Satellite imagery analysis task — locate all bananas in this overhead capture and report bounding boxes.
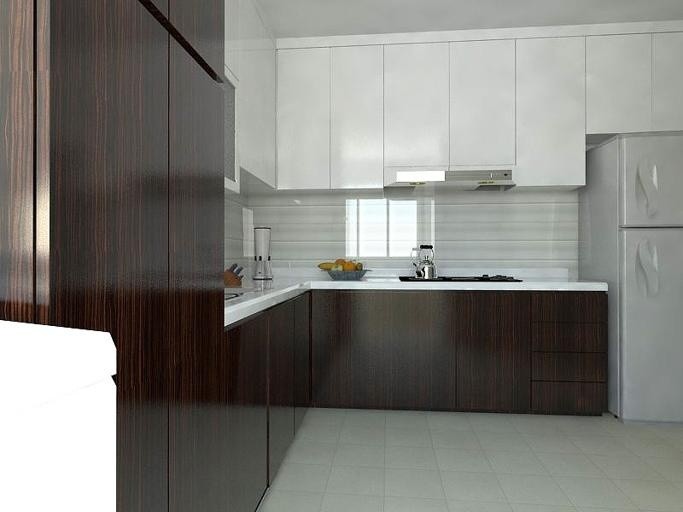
[318,263,335,270]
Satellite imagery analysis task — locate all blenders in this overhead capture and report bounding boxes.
[252,226,274,280]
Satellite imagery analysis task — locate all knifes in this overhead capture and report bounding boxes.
[228,263,244,280]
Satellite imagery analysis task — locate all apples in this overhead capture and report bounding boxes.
[344,262,353,271]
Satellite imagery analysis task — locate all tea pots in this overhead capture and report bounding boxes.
[413,244,437,280]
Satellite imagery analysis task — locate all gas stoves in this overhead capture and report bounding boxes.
[398,275,524,282]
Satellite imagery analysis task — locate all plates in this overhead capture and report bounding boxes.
[328,270,370,280]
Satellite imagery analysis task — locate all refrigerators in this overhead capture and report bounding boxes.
[576,133,683,425]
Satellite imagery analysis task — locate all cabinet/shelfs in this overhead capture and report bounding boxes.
[275,29,383,195]
[311,289,609,416]
[224,292,310,511]
[224,0,275,196]
[506,25,586,193]
[585,19,683,140]
[383,27,516,168]
[0,0,224,511]
